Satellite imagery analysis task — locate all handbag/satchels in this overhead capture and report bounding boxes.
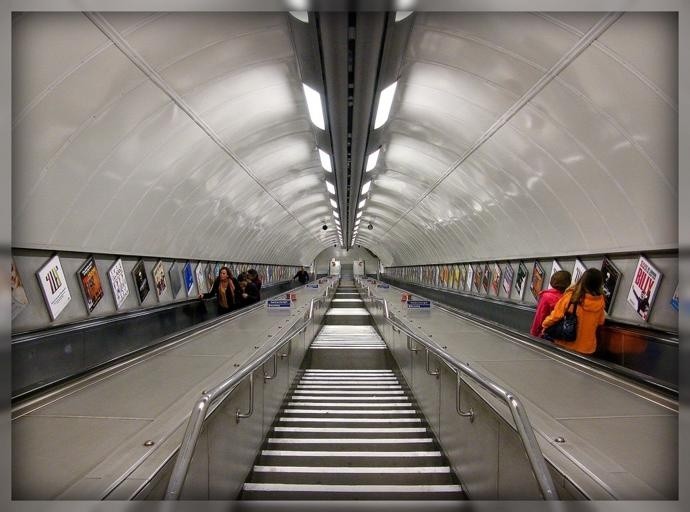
[544,312,577,341]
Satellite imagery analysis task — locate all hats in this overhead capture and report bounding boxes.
[550,271,571,289]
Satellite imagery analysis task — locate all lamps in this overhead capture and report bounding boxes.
[287,10,416,250]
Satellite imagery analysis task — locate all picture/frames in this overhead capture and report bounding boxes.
[383,253,678,327]
[12,248,308,327]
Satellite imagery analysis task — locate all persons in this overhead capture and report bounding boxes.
[529,270,572,340]
[197,267,244,316]
[631,283,651,317]
[11,258,30,305]
[539,267,609,357]
[246,269,262,302]
[235,272,260,307]
[292,266,310,285]
